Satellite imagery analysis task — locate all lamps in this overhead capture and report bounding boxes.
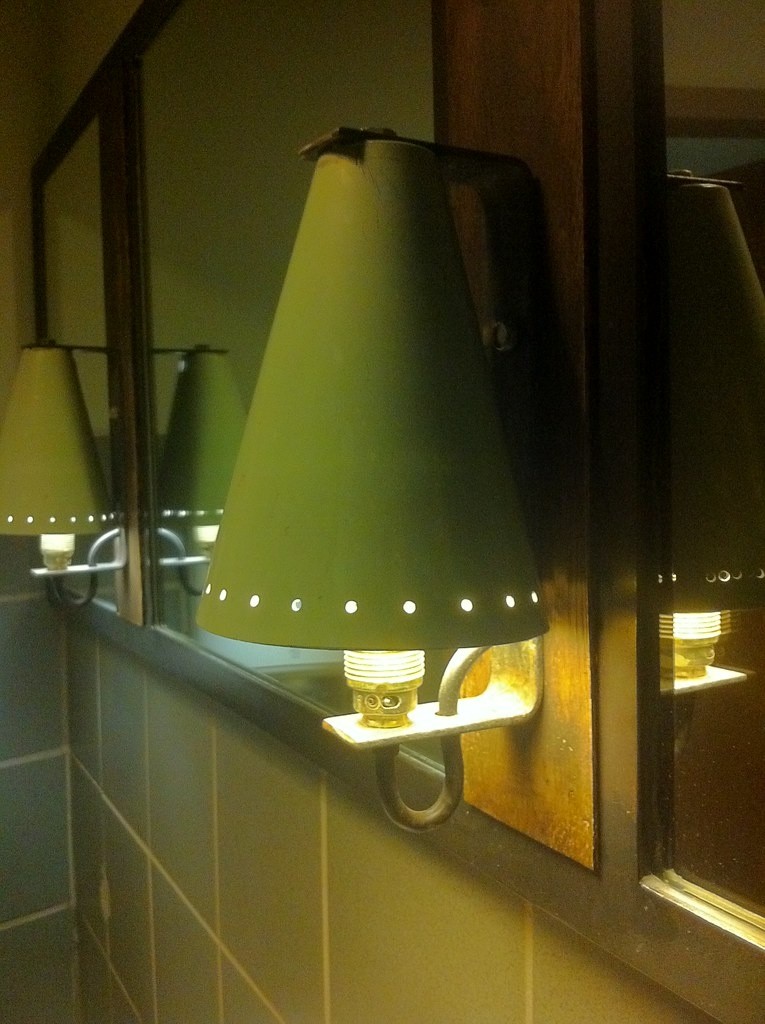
[0,338,127,609]
[156,344,248,597]
[196,124,551,835]
[658,168,765,765]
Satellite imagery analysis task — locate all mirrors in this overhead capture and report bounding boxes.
[31,0,765,1024]
